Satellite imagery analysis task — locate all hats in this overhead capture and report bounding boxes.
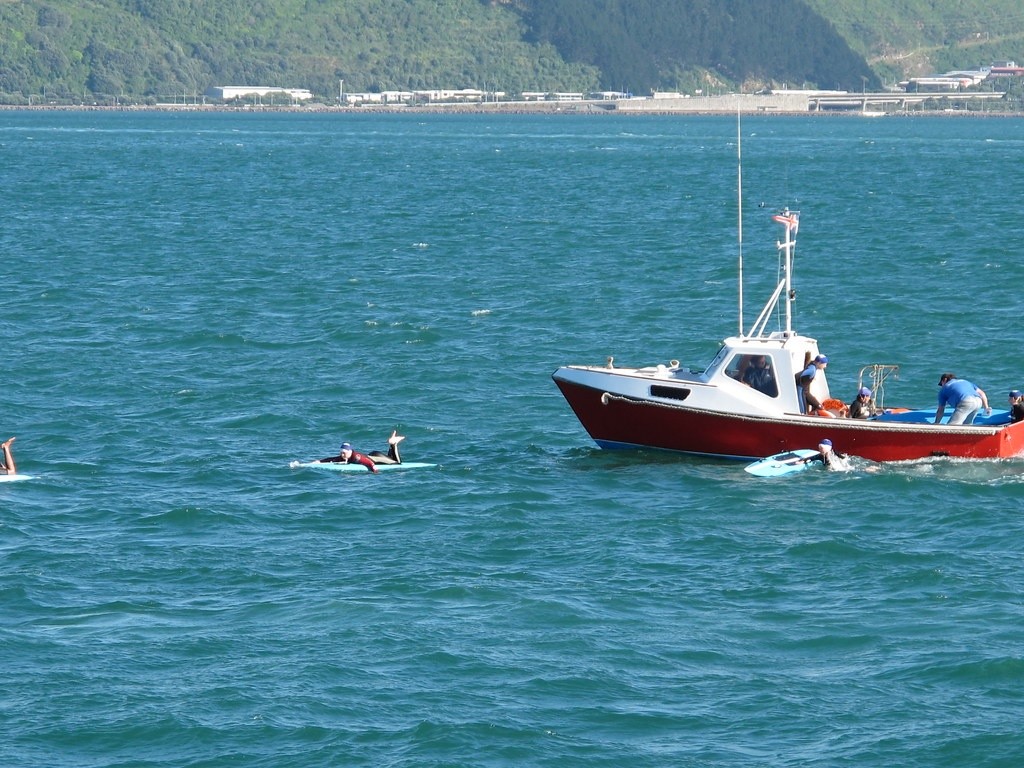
[858,386,871,396]
[1009,390,1022,397]
[815,354,828,363]
[340,443,352,452]
[938,373,950,386]
[819,439,832,450]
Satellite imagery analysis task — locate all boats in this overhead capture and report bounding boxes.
[742,449,850,477]
[549,99,1024,465]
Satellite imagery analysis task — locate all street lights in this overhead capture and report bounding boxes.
[339,79,344,104]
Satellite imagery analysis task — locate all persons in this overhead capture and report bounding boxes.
[795,438,840,468]
[0,436,18,476]
[1008,389,1024,425]
[794,353,836,417]
[848,386,885,420]
[934,373,989,426]
[310,430,405,474]
[742,354,778,398]
